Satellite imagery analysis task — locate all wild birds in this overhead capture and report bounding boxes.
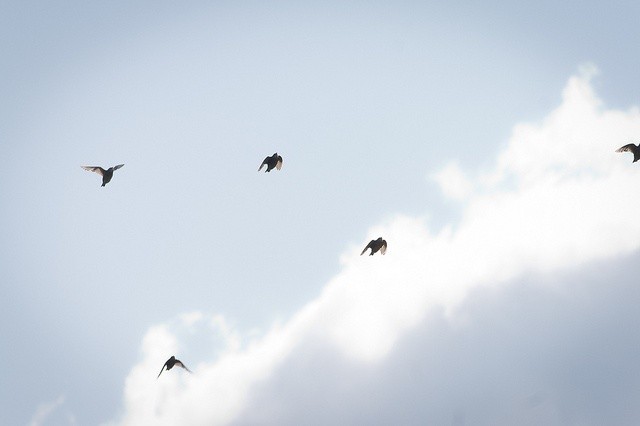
[80,164,127,187]
[615,141,640,165]
[360,236,388,257]
[157,356,192,379]
[256,151,284,174]
